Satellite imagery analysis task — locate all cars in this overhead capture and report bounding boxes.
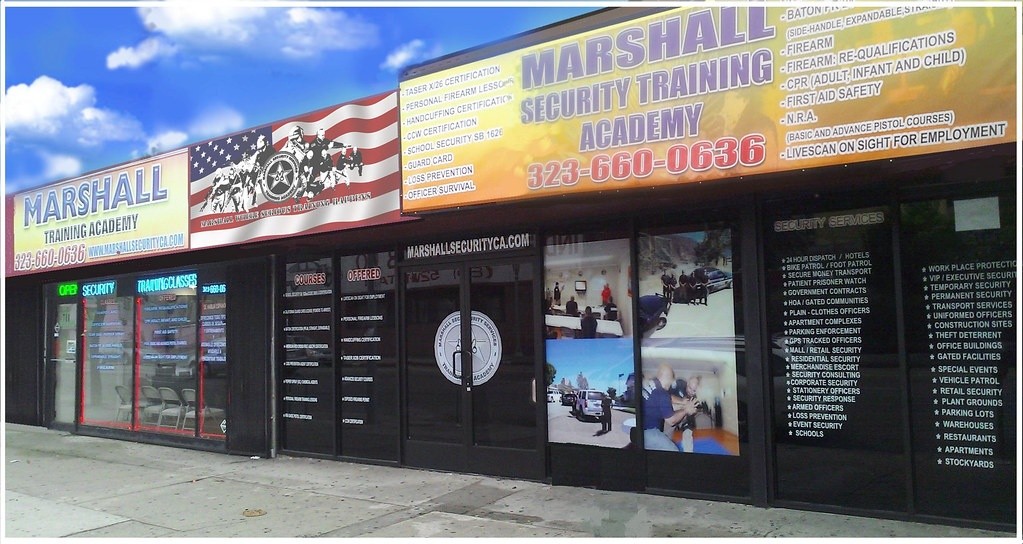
[638,293,672,332]
[189,339,227,377]
[691,267,733,293]
[287,336,317,376]
[547,387,576,405]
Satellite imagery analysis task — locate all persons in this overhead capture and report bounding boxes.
[544,279,619,339]
[600,393,612,430]
[642,363,701,452]
[660,267,711,307]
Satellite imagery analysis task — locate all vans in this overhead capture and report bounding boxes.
[574,390,605,420]
